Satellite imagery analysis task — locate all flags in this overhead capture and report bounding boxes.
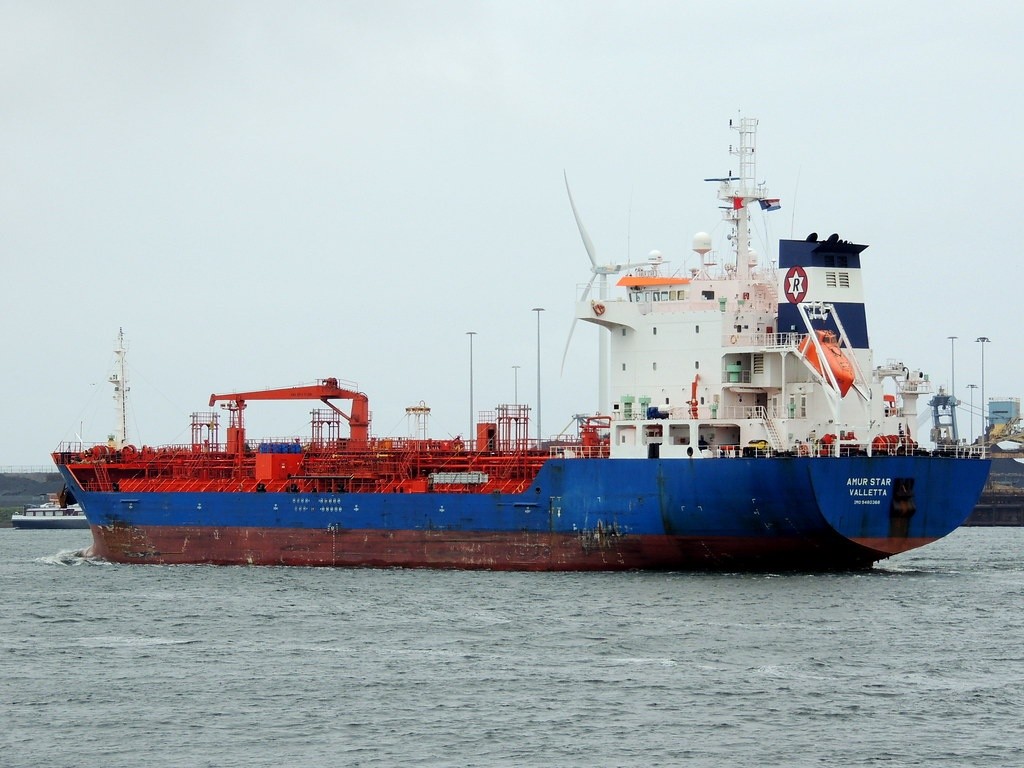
[766,199,781,211]
[734,197,744,210]
[758,200,771,210]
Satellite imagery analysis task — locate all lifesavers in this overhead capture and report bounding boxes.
[595,302,605,315]
[729,333,738,346]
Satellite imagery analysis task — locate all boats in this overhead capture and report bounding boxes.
[923,335,1024,527]
[12,502,90,530]
[49,98,993,578]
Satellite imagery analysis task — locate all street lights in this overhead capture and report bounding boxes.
[966,384,978,442]
[974,336,991,451]
[947,334,957,398]
